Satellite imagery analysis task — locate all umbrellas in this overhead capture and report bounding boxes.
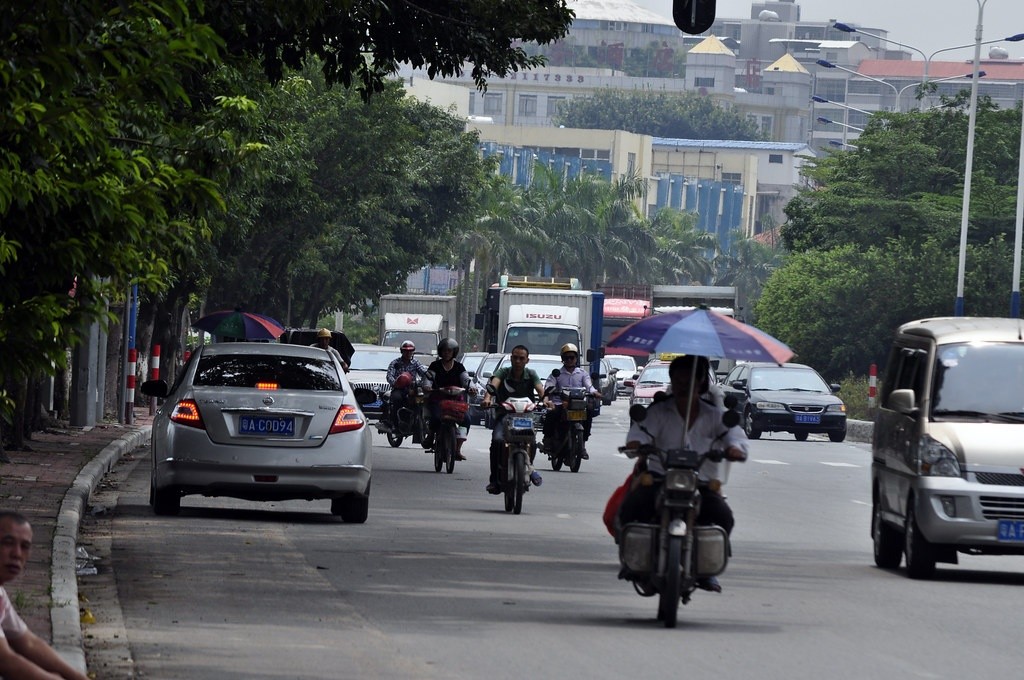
[605,302,799,450]
[189,310,286,341]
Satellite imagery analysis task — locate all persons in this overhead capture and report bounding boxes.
[0,510,92,680]
[309,328,350,372]
[386,339,427,427]
[421,337,479,460]
[603,357,725,547]
[479,345,555,494]
[542,343,602,459]
[618,356,746,593]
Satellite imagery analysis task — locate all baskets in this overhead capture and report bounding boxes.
[437,400,470,426]
[501,412,541,443]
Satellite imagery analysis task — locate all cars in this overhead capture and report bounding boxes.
[623,352,718,409]
[718,362,848,443]
[140,342,372,523]
[346,344,400,418]
[462,352,637,429]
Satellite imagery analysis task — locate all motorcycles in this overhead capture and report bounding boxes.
[617,394,747,628]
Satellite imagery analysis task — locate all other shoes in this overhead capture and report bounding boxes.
[618,567,632,578]
[486,482,499,492]
[531,472,542,486]
[582,449,589,460]
[457,450,466,460]
[700,576,721,593]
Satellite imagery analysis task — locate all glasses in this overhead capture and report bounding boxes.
[564,355,576,360]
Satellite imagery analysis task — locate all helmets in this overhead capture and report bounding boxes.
[317,328,331,337]
[400,341,416,351]
[561,343,578,358]
[438,338,459,358]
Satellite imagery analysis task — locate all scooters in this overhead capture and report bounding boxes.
[376,366,477,474]
[478,386,549,515]
[537,369,605,473]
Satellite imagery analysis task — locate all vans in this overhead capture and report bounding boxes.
[870,316,1024,579]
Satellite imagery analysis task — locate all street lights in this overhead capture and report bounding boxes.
[833,22,1024,114]
[816,60,986,113]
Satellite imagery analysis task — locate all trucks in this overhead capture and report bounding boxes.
[642,285,746,382]
[474,275,651,416]
[376,294,458,356]
